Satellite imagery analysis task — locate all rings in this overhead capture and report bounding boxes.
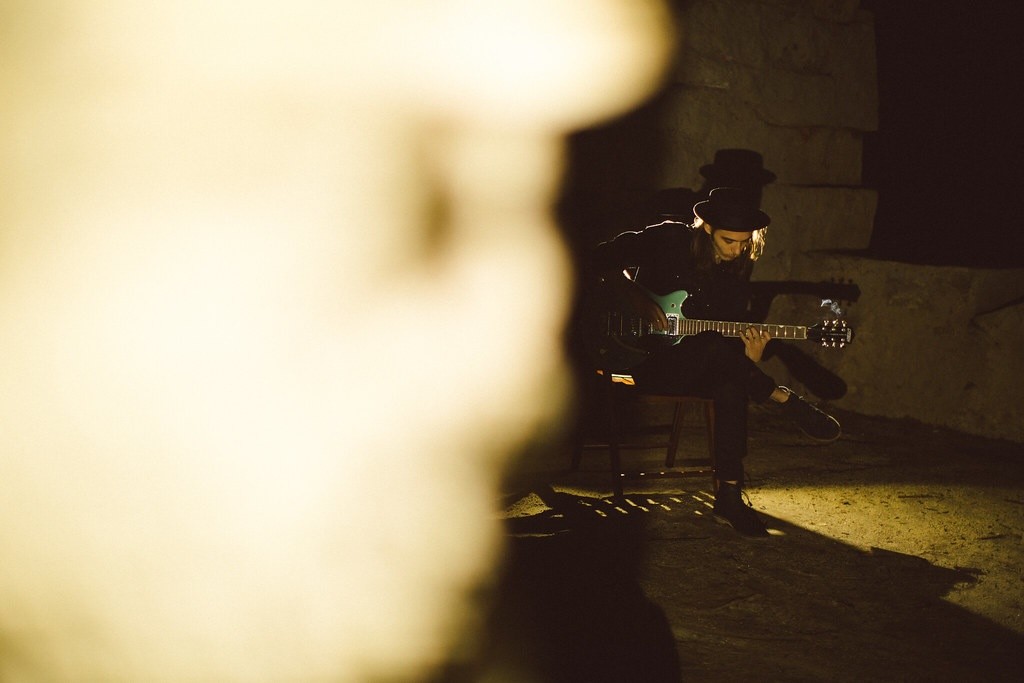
[656,317,660,322]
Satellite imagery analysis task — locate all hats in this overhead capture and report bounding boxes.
[693,187,771,232]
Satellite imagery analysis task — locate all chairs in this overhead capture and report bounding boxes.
[564,370,720,507]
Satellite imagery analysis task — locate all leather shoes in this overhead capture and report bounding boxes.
[714,482,765,531]
[777,386,842,442]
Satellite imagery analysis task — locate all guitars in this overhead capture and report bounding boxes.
[580,276,854,371]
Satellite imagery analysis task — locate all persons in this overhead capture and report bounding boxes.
[594,187,841,534]
[0,0,676,683]
